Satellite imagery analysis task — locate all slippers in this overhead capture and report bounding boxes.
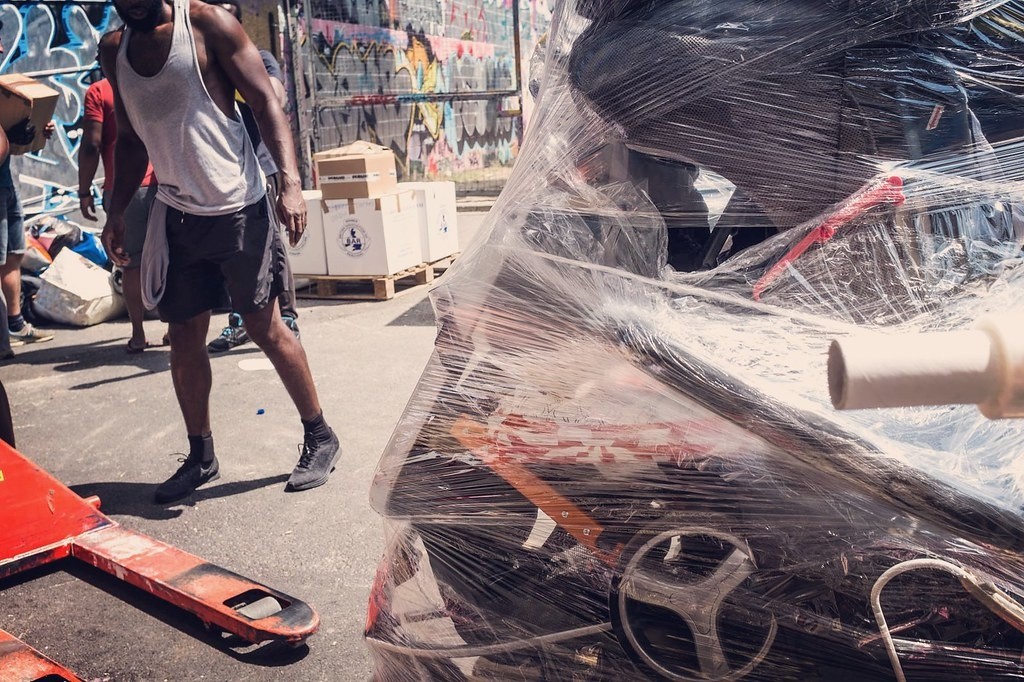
[127,337,149,353]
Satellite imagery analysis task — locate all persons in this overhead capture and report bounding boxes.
[0,18,60,368]
[100,0,341,505]
[79,0,287,349]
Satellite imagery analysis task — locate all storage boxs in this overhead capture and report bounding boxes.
[314,139,392,191]
[276,190,326,274]
[323,189,422,275]
[318,152,398,200]
[0,74,60,155]
[396,180,459,264]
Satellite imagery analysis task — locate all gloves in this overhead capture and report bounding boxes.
[5,115,37,146]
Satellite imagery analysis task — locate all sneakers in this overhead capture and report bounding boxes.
[155,454,218,504]
[8,320,53,347]
[207,313,253,354]
[284,427,342,492]
[281,316,299,333]
